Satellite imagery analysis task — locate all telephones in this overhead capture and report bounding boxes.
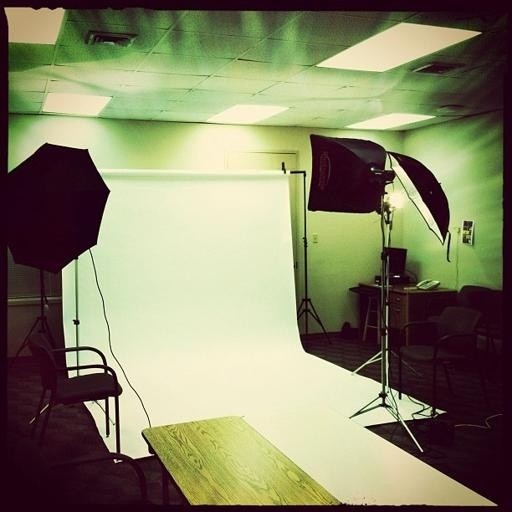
[416,279,441,291]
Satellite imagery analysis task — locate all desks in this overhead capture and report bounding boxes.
[141,417,344,507]
[359,281,458,346]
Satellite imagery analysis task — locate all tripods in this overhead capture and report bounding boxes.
[14,272,58,363]
[297,194,335,354]
[345,225,426,456]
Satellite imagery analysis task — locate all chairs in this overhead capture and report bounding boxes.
[399,285,502,385]
[28,335,122,453]
